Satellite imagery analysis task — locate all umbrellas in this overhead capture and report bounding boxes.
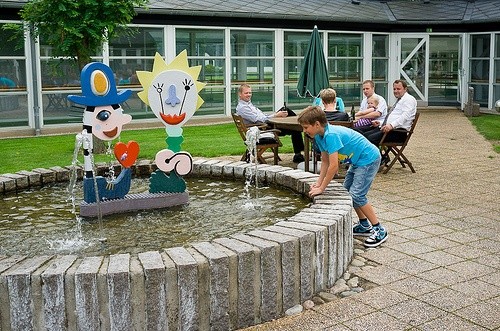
[296,25,330,99]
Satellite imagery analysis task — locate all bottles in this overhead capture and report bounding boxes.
[282,102,287,111]
[336,102,340,110]
[351,103,355,120]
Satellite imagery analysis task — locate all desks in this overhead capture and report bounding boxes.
[267,116,354,173]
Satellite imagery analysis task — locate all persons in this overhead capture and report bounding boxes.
[315,79,416,173]
[0,77,17,89]
[236,83,312,163]
[298,106,388,248]
[113,69,139,85]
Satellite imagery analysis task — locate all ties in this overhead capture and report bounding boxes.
[383,99,399,125]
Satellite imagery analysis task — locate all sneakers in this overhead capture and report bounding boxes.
[353,221,375,236]
[364,226,388,247]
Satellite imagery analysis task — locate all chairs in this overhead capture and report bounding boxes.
[231,112,283,163]
[377,112,421,175]
[302,119,353,180]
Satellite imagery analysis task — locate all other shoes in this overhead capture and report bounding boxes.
[378,156,387,171]
[293,154,304,163]
